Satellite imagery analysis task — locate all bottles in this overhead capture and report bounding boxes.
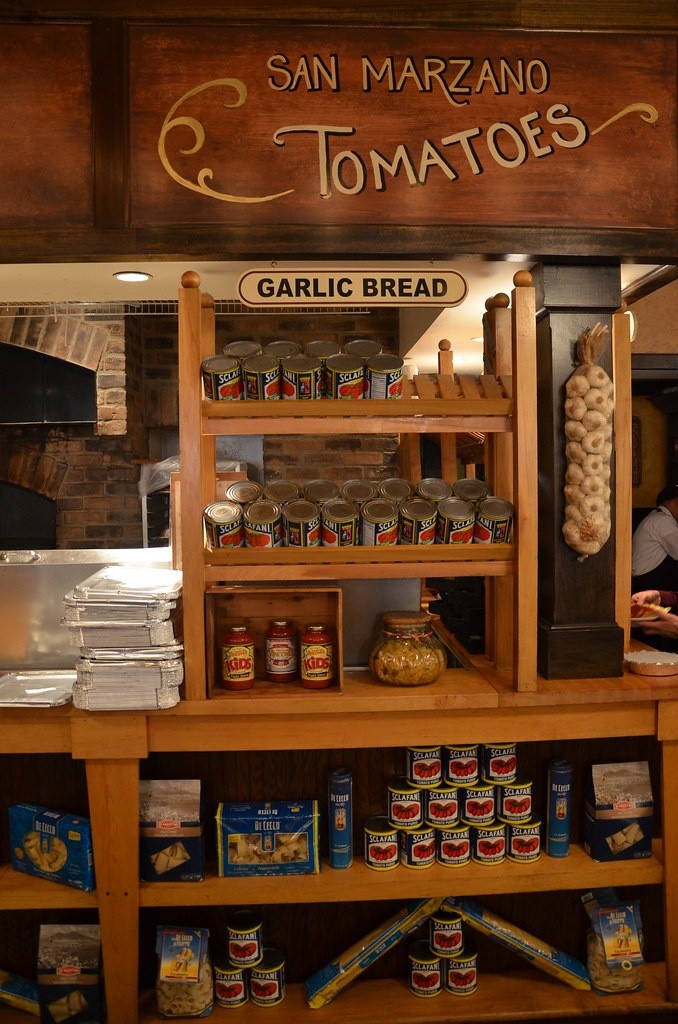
[369,611,447,686]
[543,759,573,858]
[327,766,352,868]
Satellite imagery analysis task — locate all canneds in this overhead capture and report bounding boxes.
[212,912,285,1007]
[264,620,336,688]
[201,339,404,401]
[202,477,515,548]
[220,625,254,689]
[363,741,542,871]
[407,912,477,998]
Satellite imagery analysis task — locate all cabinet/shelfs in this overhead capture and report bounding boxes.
[0,264,676,1024]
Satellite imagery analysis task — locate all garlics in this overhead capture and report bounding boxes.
[561,365,615,555]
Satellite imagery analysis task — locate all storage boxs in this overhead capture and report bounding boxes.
[9,803,95,892]
[216,801,321,877]
[582,761,653,862]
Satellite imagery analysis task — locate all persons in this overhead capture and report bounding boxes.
[631,590,678,640]
[632,486,678,594]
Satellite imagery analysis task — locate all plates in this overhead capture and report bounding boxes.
[631,606,671,627]
